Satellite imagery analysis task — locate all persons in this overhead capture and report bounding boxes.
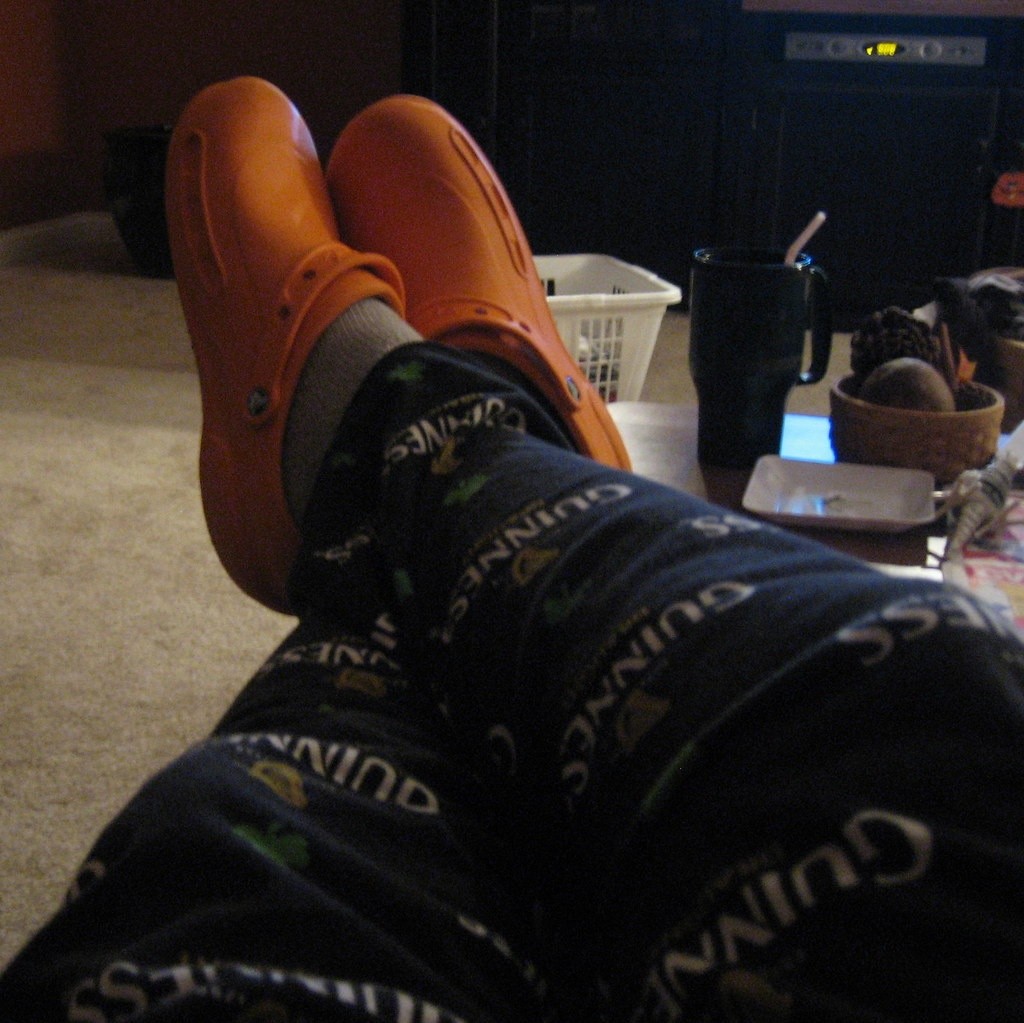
[0,77,1024,1022]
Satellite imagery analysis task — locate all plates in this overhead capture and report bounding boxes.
[741,454,935,534]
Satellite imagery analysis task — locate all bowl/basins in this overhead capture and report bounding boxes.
[827,371,1007,489]
[932,310,1024,436]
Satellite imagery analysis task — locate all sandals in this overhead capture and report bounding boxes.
[324,93,631,474]
[163,74,407,616]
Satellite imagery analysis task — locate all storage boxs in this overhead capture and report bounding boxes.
[533,254,682,408]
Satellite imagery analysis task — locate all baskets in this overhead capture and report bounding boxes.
[530,253,682,402]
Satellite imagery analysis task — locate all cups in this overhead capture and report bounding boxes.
[687,245,833,469]
